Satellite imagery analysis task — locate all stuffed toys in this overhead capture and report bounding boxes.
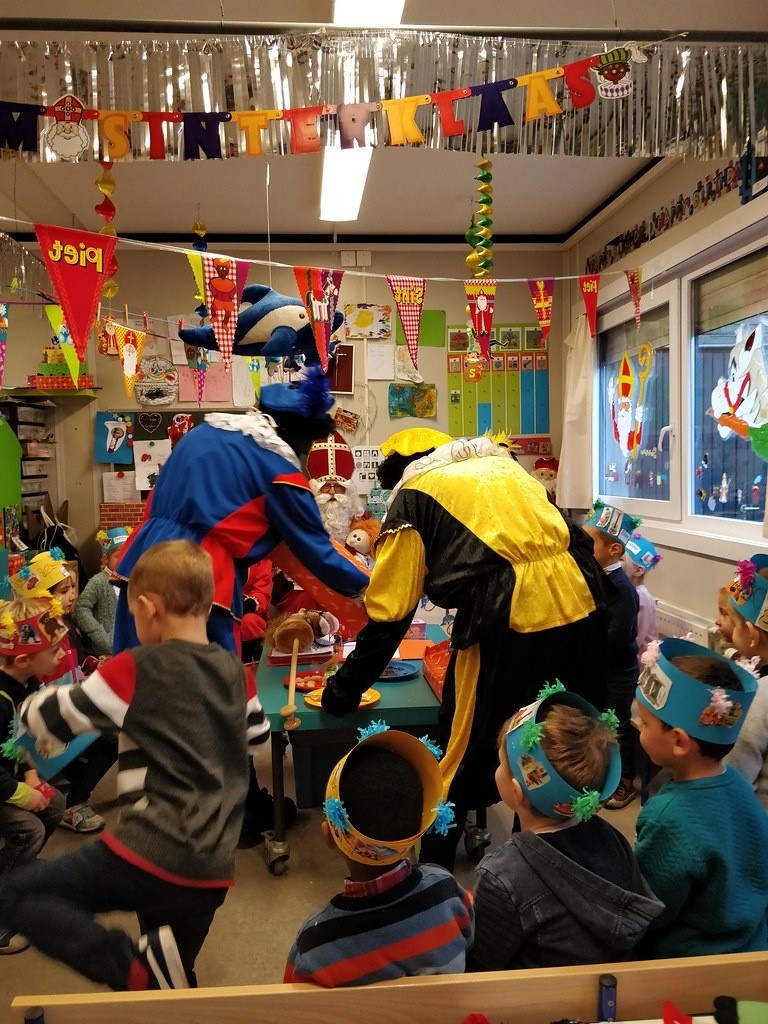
[342,515,384,568]
[178,282,345,357]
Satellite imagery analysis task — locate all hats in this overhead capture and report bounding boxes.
[255,362,336,417]
[379,428,456,456]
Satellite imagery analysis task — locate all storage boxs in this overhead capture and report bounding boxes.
[16,406,54,531]
[27,345,94,390]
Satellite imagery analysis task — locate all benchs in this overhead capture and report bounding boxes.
[9,952,768,1024]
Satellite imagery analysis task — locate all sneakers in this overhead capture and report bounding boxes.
[60,800,106,832]
[605,781,638,808]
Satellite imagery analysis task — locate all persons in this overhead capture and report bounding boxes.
[712,552,768,812]
[627,637,768,962]
[110,366,369,851]
[0,546,122,954]
[577,499,666,807]
[281,719,476,988]
[75,527,134,658]
[1,538,275,992]
[462,678,666,973]
[315,427,611,873]
[531,454,560,509]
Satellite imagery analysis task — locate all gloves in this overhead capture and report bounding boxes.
[320,686,361,718]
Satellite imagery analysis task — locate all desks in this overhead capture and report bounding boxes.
[254,622,442,874]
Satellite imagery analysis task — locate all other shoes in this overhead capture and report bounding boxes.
[238,788,300,851]
[136,923,189,989]
[0,928,30,954]
